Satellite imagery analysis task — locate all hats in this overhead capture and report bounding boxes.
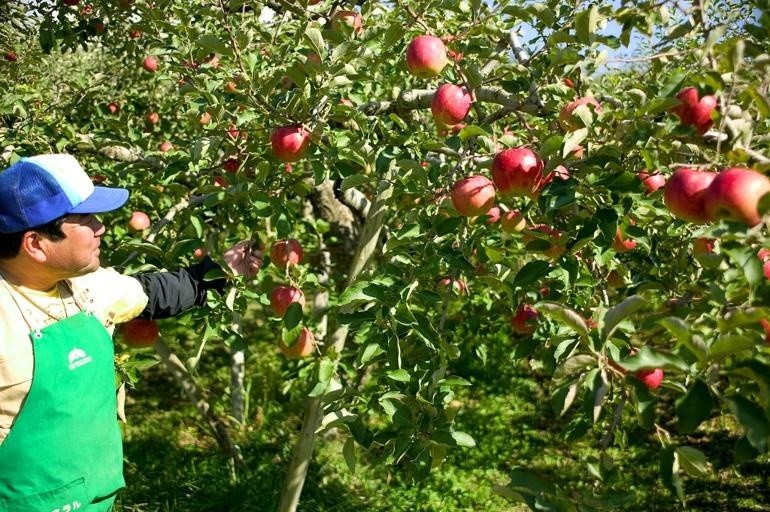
[0,153,130,233]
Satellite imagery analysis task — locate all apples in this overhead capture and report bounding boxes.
[407,33,770,389]
[3,1,365,363]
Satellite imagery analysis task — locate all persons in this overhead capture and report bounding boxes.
[0,152,267,512]
